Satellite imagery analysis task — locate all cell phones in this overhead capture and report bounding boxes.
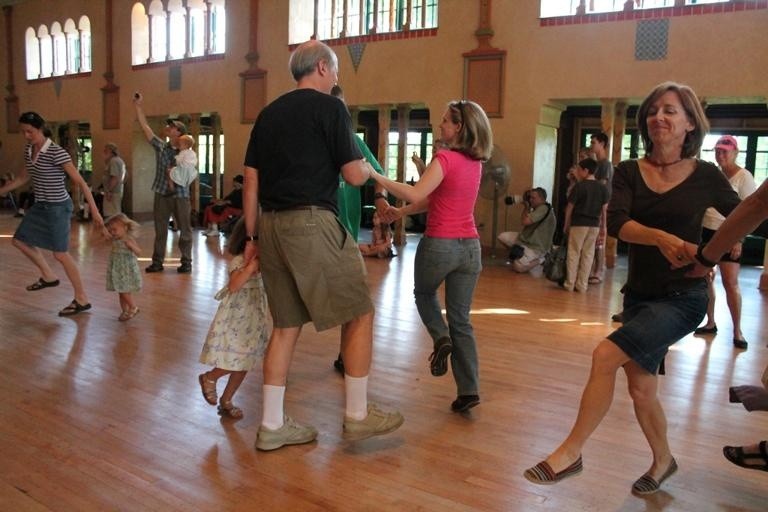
[135,93,139,99]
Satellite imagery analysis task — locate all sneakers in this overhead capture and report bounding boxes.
[430,338,453,376]
[177,266,192,273]
[734,329,747,348]
[342,404,404,441]
[451,394,479,413]
[523,453,584,486]
[146,264,163,273]
[694,326,717,333]
[202,229,218,237]
[255,414,318,450]
[631,456,677,497]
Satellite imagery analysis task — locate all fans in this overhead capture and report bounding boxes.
[478,141,513,269]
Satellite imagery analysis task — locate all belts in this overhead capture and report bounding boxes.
[262,205,329,212]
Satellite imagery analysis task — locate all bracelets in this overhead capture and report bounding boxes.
[246,236,258,241]
[374,191,388,200]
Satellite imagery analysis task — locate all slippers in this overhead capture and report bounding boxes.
[59,300,91,316]
[27,278,59,290]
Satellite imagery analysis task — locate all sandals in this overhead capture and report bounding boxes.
[723,441,767,471]
[118,308,140,321]
[199,372,217,404]
[217,397,242,417]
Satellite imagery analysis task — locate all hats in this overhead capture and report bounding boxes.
[106,144,119,154]
[712,136,738,150]
[233,175,243,184]
[167,119,186,133]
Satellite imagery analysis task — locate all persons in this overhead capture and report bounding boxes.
[76,185,104,225]
[359,212,395,258]
[90,212,141,321]
[133,91,198,273]
[329,86,395,376]
[362,100,492,413]
[496,134,615,294]
[670,135,768,471]
[202,175,243,236]
[103,144,126,221]
[524,83,745,495]
[244,39,404,451]
[1,111,111,317]
[198,215,273,418]
[14,191,34,217]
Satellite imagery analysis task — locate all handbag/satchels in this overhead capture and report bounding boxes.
[543,246,566,281]
[509,245,524,260]
